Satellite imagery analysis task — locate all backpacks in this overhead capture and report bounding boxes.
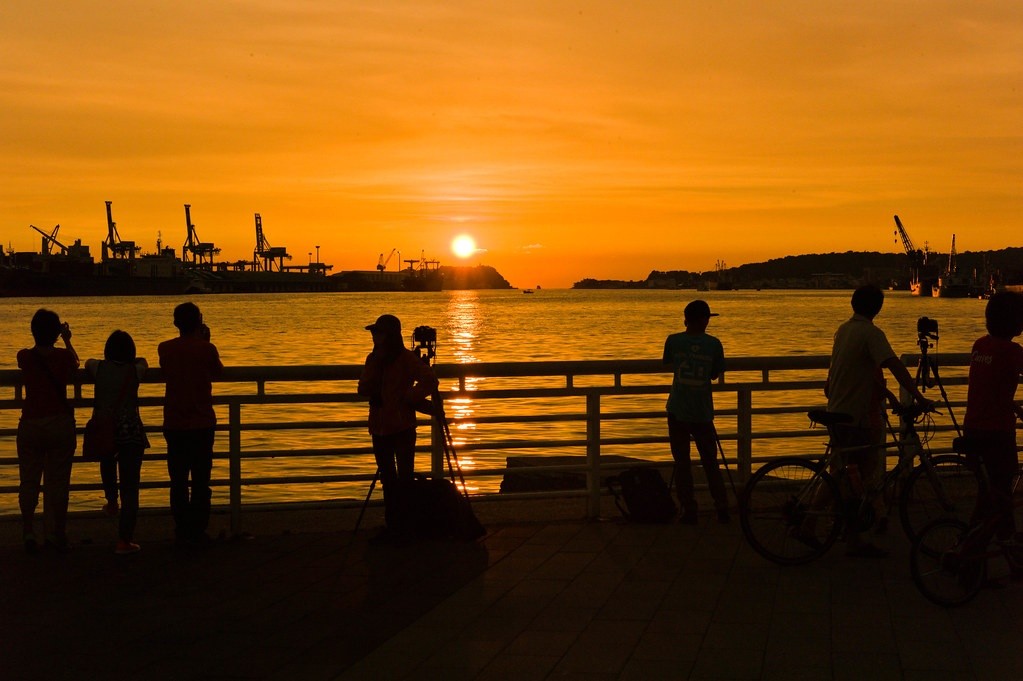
[413,475,487,540]
[605,467,678,522]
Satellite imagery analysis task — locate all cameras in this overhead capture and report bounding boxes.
[413,326,437,342]
[917,317,938,332]
[60,323,66,333]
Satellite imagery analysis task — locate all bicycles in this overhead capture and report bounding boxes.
[740,401,1023,609]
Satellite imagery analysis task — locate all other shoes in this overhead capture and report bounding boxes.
[25,540,37,552]
[175,532,210,546]
[718,513,731,524]
[848,542,892,560]
[115,542,141,555]
[679,507,698,523]
[102,502,119,517]
[44,536,67,549]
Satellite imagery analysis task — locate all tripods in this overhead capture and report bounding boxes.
[913,332,962,437]
[352,345,470,538]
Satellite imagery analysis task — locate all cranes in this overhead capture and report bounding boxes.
[947,234,958,273]
[182,204,221,265]
[379,247,397,268]
[253,213,291,272]
[894,214,915,254]
[102,200,142,259]
[30,225,71,254]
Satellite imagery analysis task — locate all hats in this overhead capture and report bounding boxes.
[365,315,401,332]
[685,301,720,319]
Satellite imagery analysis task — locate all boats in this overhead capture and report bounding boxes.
[523,289,534,293]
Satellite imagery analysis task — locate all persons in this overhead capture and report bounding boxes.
[357,314,440,515]
[958,290,1023,591]
[157,301,225,508]
[663,300,734,524]
[82,330,151,510]
[16,309,80,515]
[825,283,938,557]
